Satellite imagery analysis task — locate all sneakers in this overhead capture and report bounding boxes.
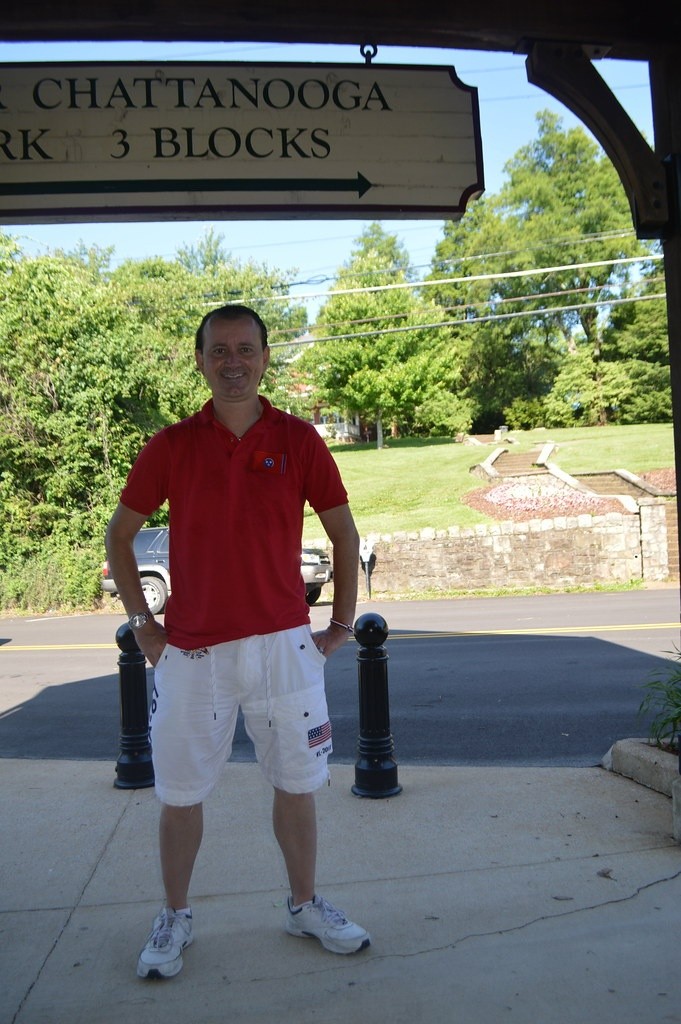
[136,906,194,980]
[285,892,372,954]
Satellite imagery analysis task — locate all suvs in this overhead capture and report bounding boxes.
[101,526,334,616]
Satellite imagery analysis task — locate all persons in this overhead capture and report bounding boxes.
[104,304,371,979]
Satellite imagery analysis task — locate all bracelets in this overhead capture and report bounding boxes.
[330,619,353,632]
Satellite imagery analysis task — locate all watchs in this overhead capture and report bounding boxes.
[128,612,154,630]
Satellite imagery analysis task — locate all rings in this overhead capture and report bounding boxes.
[318,648,324,653]
